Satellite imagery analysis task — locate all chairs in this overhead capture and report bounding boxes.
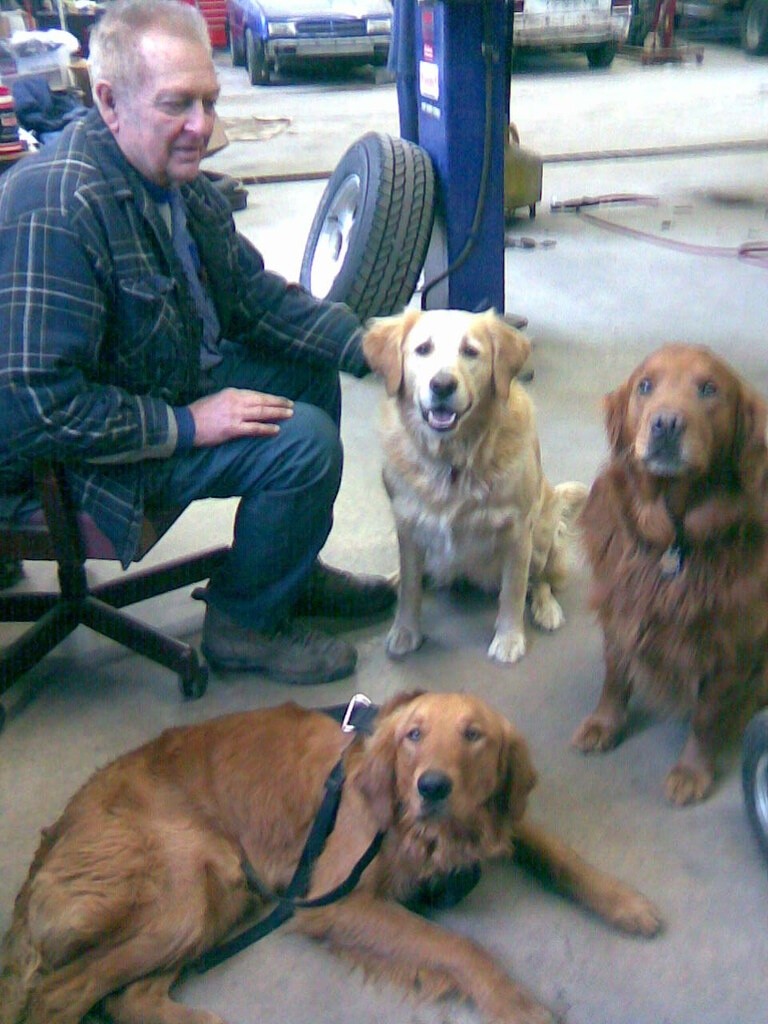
[0,441,237,730]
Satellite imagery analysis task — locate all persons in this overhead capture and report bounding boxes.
[1,1,395,686]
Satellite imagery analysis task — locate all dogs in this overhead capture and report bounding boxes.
[359,304,768,808]
[0,685,668,1024]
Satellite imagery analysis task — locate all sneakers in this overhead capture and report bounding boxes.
[191,586,357,683]
[289,560,399,629]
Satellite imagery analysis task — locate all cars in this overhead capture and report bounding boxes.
[419,0,633,67]
[225,0,396,86]
